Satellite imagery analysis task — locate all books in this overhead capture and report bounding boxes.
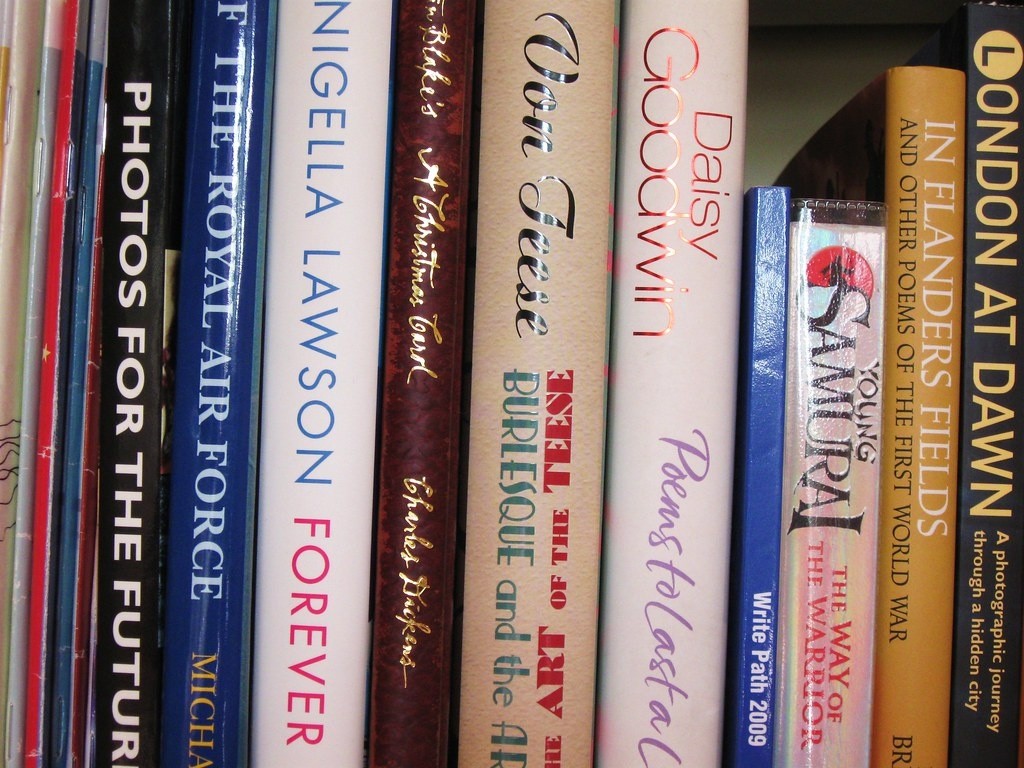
[1,1,1024,768]
